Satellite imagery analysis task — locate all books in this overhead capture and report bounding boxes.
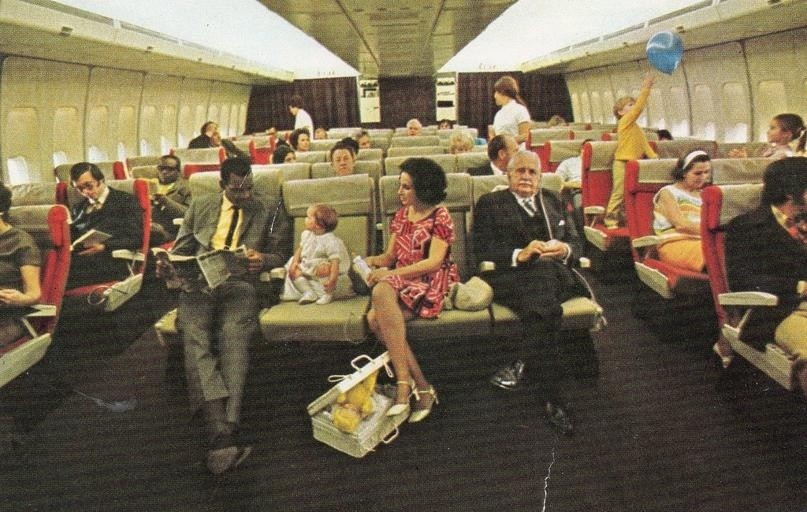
[70,230,115,253]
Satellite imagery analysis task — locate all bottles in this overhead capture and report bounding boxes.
[351,252,374,288]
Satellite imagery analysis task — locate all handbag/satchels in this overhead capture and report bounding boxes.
[282,256,357,301]
[443,275,494,312]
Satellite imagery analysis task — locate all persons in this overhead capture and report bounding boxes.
[349,158,461,424]
[188,95,477,176]
[63,165,141,289]
[652,110,806,398]
[289,203,348,306]
[150,156,288,474]
[473,77,596,437]
[0,184,43,350]
[606,77,658,233]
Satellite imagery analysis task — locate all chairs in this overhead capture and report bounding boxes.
[694,185,797,387]
[153,122,601,383]
[582,126,777,312]
[8,150,166,333]
[2,205,67,390]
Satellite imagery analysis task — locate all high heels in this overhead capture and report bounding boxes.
[385,377,420,416]
[407,384,440,423]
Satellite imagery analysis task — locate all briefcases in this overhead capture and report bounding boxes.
[307,351,410,458]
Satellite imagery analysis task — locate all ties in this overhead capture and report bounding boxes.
[224,205,240,250]
[523,200,536,215]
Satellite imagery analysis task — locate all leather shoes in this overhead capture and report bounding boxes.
[543,401,573,435]
[205,436,252,474]
[490,360,531,392]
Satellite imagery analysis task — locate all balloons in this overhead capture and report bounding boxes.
[647,31,684,77]
